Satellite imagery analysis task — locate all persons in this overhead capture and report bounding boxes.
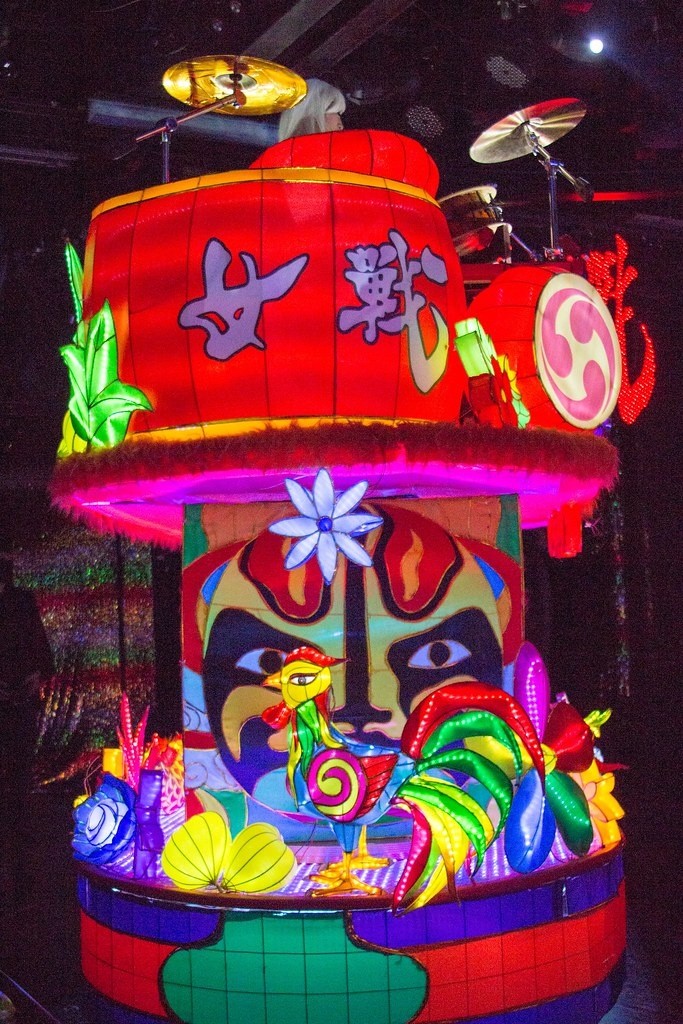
[277,78,347,143]
[0,557,55,819]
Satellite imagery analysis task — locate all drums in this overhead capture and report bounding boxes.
[436,184,512,259]
[465,264,623,435]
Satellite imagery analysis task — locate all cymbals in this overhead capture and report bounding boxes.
[469,96,587,166]
[162,53,308,117]
